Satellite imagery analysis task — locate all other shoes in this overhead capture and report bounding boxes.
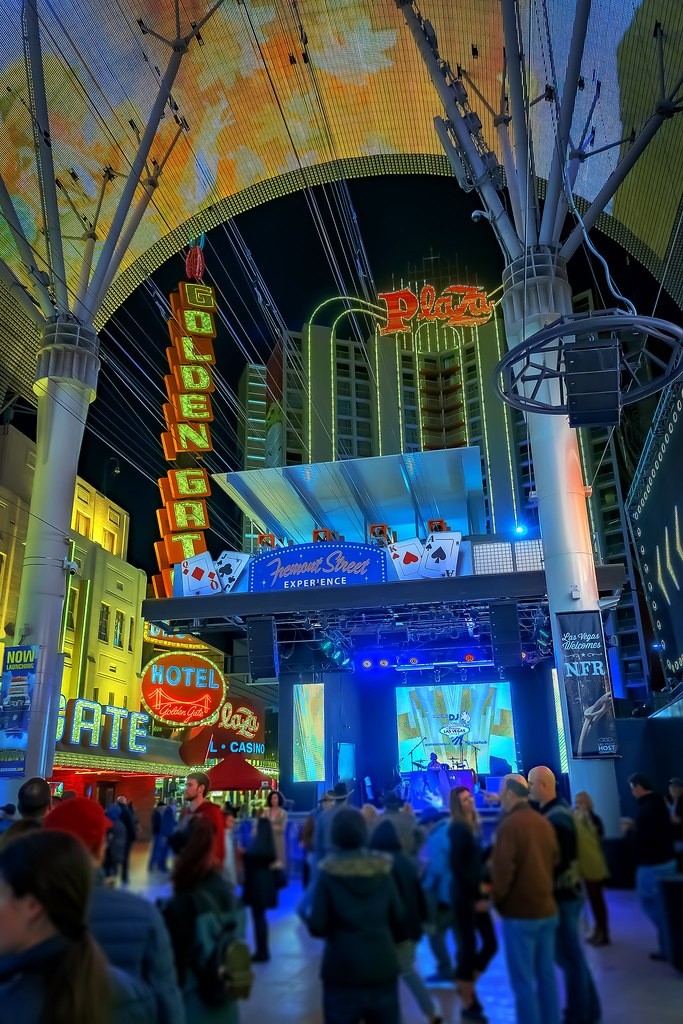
[457,988,483,1013]
[426,963,455,983]
[432,1015,443,1024]
[650,952,670,962]
[463,1004,487,1024]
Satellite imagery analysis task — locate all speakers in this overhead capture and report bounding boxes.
[564,338,623,428]
[489,601,522,668]
[246,615,279,681]
[485,777,504,795]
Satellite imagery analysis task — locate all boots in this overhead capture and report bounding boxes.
[584,922,610,947]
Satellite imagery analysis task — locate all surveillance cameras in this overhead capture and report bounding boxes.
[69,563,78,575]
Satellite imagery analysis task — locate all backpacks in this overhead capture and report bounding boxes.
[194,879,253,1001]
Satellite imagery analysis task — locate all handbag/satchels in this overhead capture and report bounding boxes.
[274,868,289,889]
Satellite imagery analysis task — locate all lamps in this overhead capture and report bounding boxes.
[300,609,482,667]
[537,627,551,649]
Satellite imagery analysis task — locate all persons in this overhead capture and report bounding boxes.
[0,752,681,1021]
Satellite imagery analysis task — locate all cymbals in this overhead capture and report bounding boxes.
[411,762,426,769]
[414,760,425,762]
[446,757,459,761]
[452,763,462,765]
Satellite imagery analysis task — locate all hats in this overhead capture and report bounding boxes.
[327,783,354,799]
[382,792,402,808]
[418,806,442,825]
[44,796,112,856]
[319,794,335,803]
[157,802,166,807]
